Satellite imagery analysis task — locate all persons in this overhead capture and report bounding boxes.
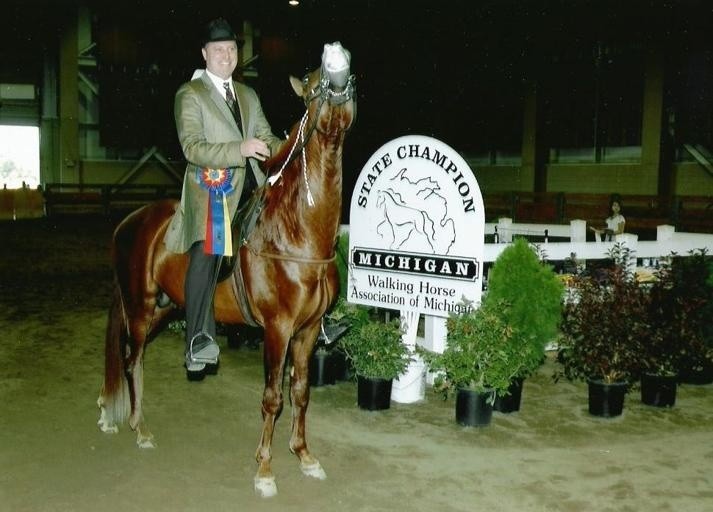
[590,198,625,241]
[174,17,354,372]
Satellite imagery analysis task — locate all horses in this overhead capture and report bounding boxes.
[96,38,362,500]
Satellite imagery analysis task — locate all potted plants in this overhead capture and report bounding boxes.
[338,317,416,410]
[298,230,371,388]
[550,240,646,418]
[633,269,709,407]
[442,235,566,429]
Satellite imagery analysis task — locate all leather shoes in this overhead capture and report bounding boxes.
[186,340,219,373]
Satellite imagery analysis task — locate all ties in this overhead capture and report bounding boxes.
[223,83,236,113]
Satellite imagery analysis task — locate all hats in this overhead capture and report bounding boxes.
[200,20,234,44]
